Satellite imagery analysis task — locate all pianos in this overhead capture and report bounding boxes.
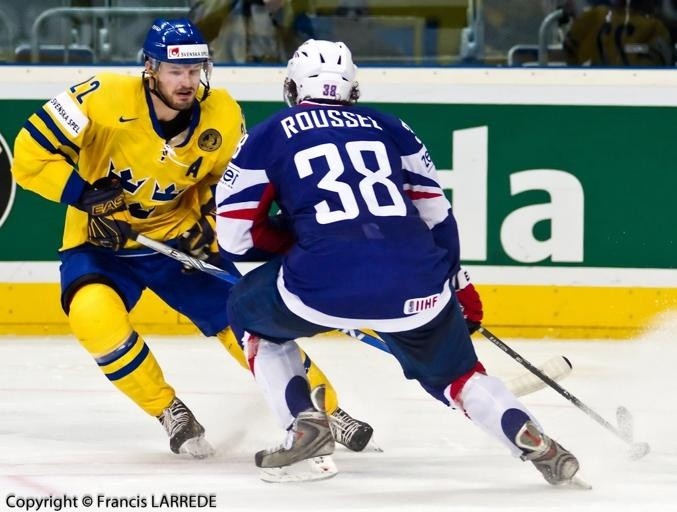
[477,326,633,444]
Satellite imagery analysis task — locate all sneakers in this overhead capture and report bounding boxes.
[156,397,206,455]
[328,407,375,453]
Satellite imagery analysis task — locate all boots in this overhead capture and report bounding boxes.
[515,420,579,487]
[254,383,336,469]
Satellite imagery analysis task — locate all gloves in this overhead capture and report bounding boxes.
[83,175,132,254]
[179,206,218,273]
[452,267,485,336]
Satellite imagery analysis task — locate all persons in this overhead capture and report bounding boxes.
[213,38,579,487]
[10,16,374,455]
[187,0,315,64]
[559,1,676,69]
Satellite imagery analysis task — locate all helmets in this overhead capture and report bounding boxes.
[282,39,361,107]
[143,16,213,83]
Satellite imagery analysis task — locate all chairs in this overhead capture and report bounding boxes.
[0,0,677,68]
